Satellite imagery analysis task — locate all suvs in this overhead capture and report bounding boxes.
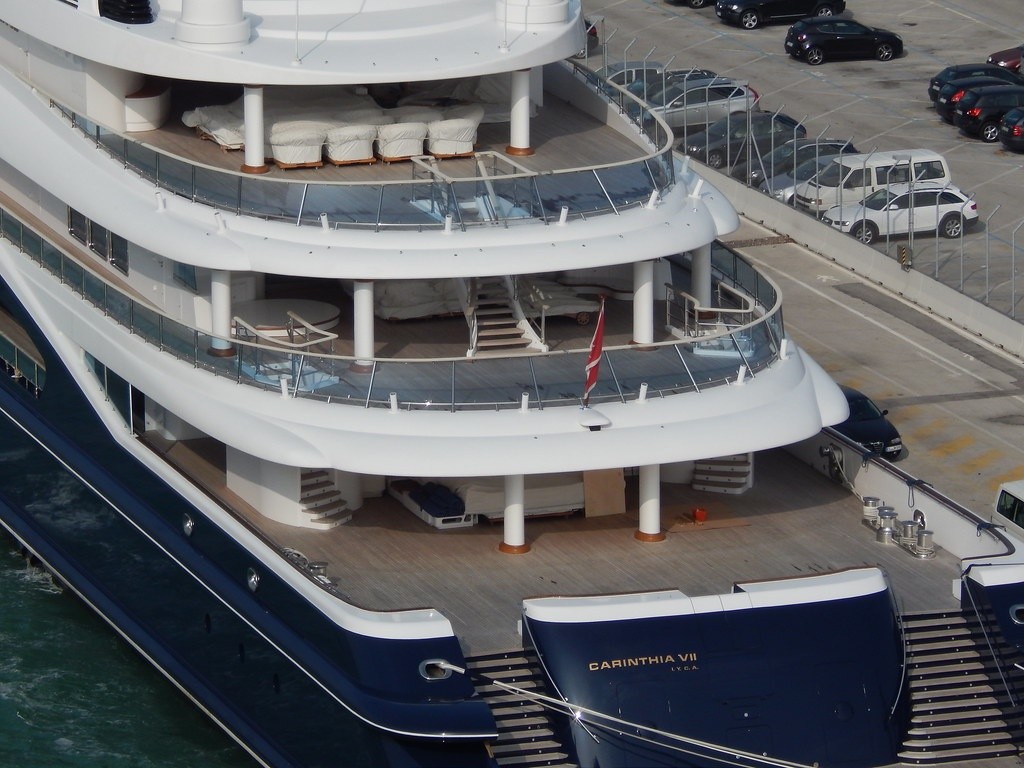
[625,74,762,134]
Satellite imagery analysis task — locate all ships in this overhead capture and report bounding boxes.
[3,0,1024,768]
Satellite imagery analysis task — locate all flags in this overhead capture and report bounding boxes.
[582,299,605,399]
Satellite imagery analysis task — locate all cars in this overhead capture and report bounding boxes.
[986,42,1024,73]
[937,76,1019,124]
[615,68,718,109]
[714,0,847,29]
[758,153,860,208]
[677,108,809,170]
[927,62,1023,104]
[822,178,978,244]
[954,85,1023,141]
[664,0,719,8]
[730,135,856,191]
[826,383,903,460]
[591,58,666,94]
[996,103,1024,154]
[570,20,598,59]
[783,15,904,63]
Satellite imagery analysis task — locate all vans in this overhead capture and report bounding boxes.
[992,479,1024,541]
[795,148,953,219]
